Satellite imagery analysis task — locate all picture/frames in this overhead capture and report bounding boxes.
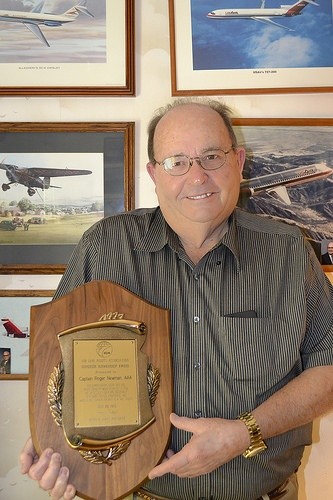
[169,0,333,96]
[218,118,333,272]
[0,122,135,273]
[0,290,60,381]
[0,0,136,97]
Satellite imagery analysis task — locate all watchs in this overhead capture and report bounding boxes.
[237,412,267,458]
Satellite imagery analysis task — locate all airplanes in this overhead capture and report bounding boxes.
[0,3,90,46]
[209,1,317,28]
[0,162,92,196]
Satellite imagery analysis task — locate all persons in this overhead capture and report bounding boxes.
[19,98,333,500]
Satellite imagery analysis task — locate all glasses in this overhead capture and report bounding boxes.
[152,145,234,176]
